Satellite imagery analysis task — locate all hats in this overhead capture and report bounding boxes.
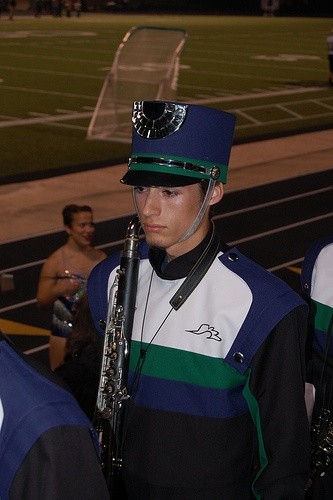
[120,101,237,187]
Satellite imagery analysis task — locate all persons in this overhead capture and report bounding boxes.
[85,101,311,500]
[37,204,109,375]
